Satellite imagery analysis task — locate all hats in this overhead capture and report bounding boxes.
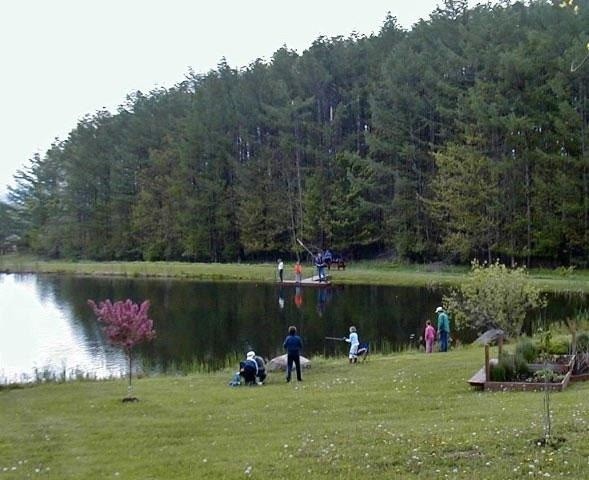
[434,306,443,313]
[246,351,255,360]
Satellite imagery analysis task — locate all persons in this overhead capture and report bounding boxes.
[295,261,303,283]
[315,247,343,281]
[239,348,268,385]
[343,326,361,364]
[278,288,285,309]
[277,258,284,282]
[424,305,451,353]
[284,326,306,381]
[317,286,344,318]
[295,286,304,308]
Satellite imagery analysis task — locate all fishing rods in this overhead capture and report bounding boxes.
[325,337,346,342]
[296,238,321,264]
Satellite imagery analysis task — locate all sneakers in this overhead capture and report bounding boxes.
[348,361,357,366]
[439,349,447,352]
